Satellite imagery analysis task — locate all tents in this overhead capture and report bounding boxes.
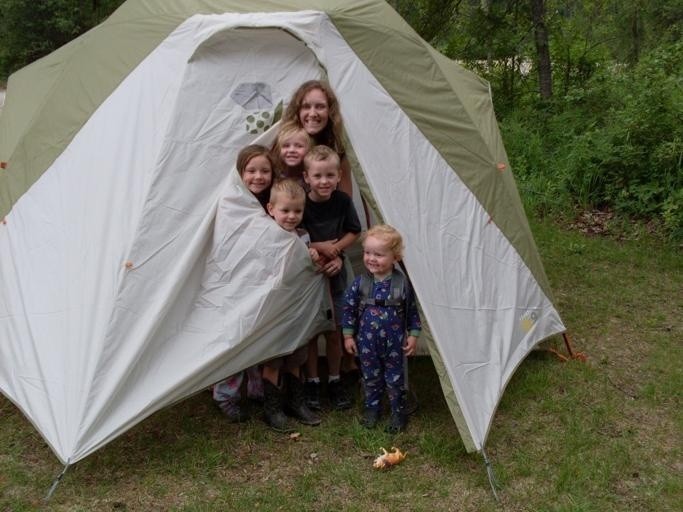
[0,0,575,505]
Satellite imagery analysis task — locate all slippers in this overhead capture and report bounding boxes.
[340,368,361,382]
[218,400,240,417]
[246,393,264,401]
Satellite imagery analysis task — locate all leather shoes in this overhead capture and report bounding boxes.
[329,379,353,408]
[305,381,321,408]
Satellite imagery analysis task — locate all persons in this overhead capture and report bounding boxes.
[261,178,324,433]
[283,79,363,387]
[338,223,422,435]
[212,143,275,424]
[271,124,312,184]
[301,144,354,413]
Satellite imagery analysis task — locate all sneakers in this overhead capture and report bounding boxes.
[384,412,407,433]
[363,409,378,428]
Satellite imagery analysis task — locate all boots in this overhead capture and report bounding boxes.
[282,376,322,424]
[262,378,290,432]
